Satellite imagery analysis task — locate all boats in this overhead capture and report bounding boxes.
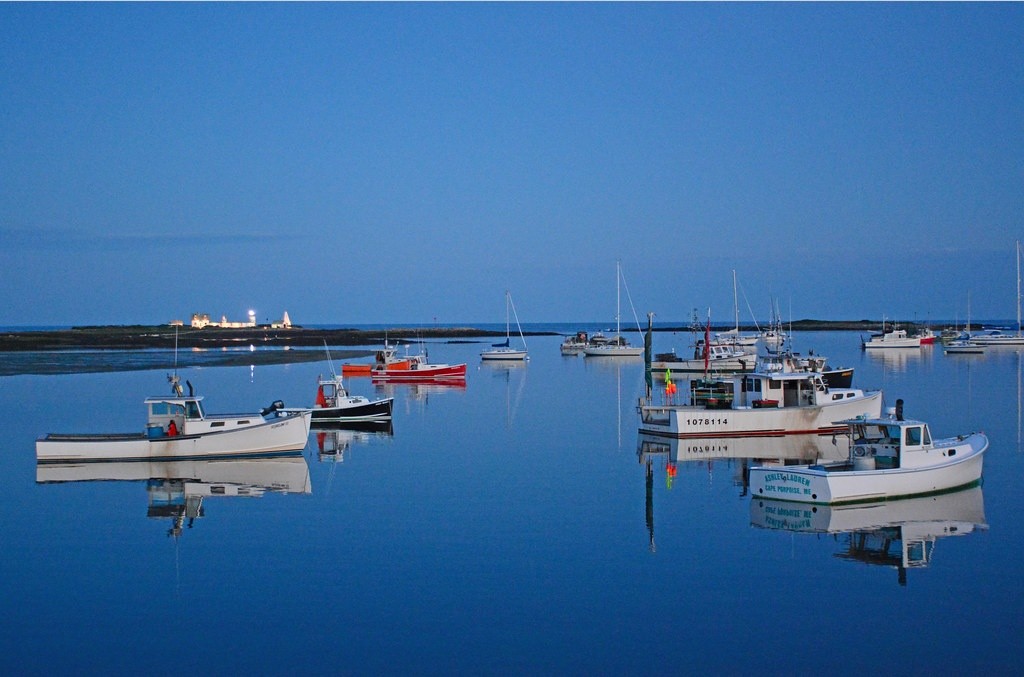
[342,371,467,405]
[370,343,466,378]
[309,418,395,464]
[651,345,756,372]
[635,367,883,437]
[635,431,850,554]
[749,483,993,586]
[275,339,394,420]
[35,459,312,541]
[560,341,585,355]
[942,341,988,353]
[748,398,989,505]
[482,360,529,419]
[341,329,420,372]
[589,332,608,344]
[920,326,938,344]
[940,327,962,343]
[860,329,921,348]
[756,346,829,374]
[35,323,313,461]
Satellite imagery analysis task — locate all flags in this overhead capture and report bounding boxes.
[645,332,652,388]
[705,320,710,368]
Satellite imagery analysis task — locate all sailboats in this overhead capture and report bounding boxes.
[736,294,786,340]
[481,289,528,360]
[583,260,645,355]
[967,240,1024,345]
[709,269,762,345]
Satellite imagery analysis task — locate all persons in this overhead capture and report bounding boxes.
[167,420,176,436]
